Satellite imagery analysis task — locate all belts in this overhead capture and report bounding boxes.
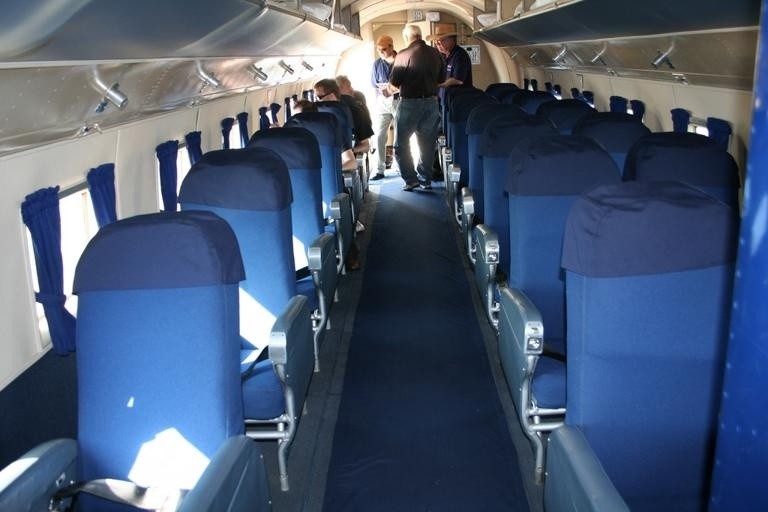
[402,94,436,97]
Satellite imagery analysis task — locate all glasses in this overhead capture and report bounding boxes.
[377,48,387,50]
[316,92,332,101]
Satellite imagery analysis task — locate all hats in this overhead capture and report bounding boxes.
[376,35,392,46]
[426,24,460,41]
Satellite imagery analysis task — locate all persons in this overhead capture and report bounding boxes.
[314,78,374,152]
[336,76,371,127]
[369,25,473,192]
[270,99,358,171]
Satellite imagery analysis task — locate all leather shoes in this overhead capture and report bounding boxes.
[369,174,384,180]
[386,163,391,168]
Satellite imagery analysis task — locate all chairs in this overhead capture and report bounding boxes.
[0,209,274,511]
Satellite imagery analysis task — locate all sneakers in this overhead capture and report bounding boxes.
[420,183,431,189]
[402,181,420,190]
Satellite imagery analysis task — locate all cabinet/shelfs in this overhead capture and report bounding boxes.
[472,0,565,36]
[270,0,361,34]
